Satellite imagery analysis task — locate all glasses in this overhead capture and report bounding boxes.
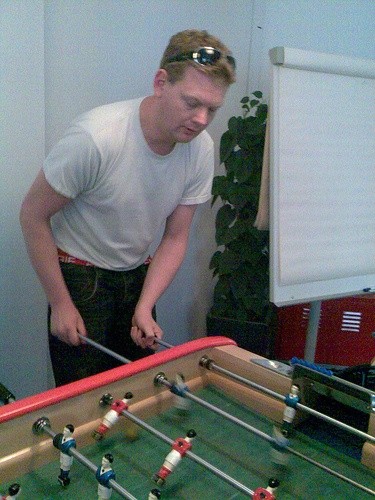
[160,46,236,71]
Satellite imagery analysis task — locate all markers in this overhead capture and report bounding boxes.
[363,288,375,292]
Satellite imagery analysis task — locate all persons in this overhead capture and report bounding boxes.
[58,424,77,487]
[19,28,237,388]
[0,484,22,500]
[91,392,133,440]
[252,476,280,500]
[281,384,300,437]
[170,373,190,420]
[152,430,196,486]
[148,488,161,500]
[96,453,116,500]
[271,426,290,475]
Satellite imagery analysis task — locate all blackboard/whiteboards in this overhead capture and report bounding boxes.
[268,48,375,309]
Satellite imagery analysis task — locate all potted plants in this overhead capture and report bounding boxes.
[205,90,278,361]
